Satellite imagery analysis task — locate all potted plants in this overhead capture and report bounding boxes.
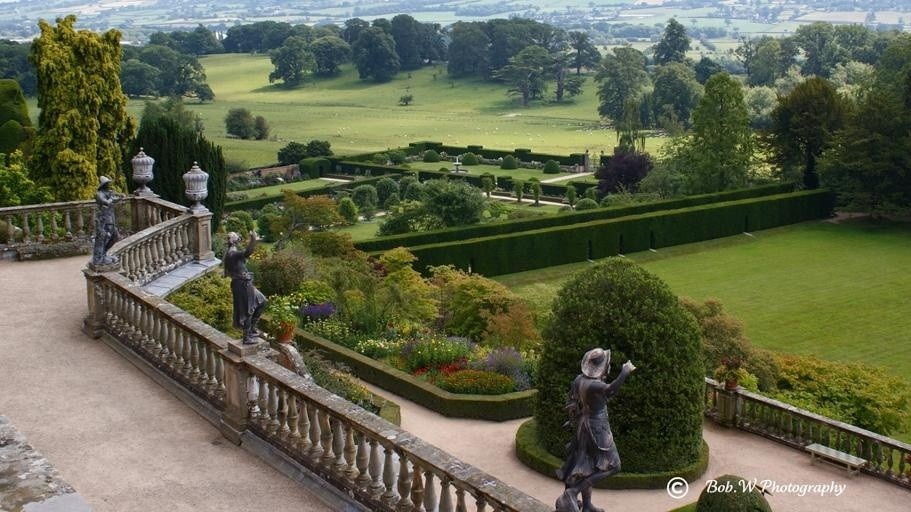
[725,368,746,391]
[263,293,309,343]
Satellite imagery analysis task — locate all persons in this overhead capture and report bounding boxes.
[557,346,636,512]
[90,174,124,264]
[219,228,273,344]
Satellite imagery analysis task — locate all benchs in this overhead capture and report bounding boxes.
[805,442,868,479]
